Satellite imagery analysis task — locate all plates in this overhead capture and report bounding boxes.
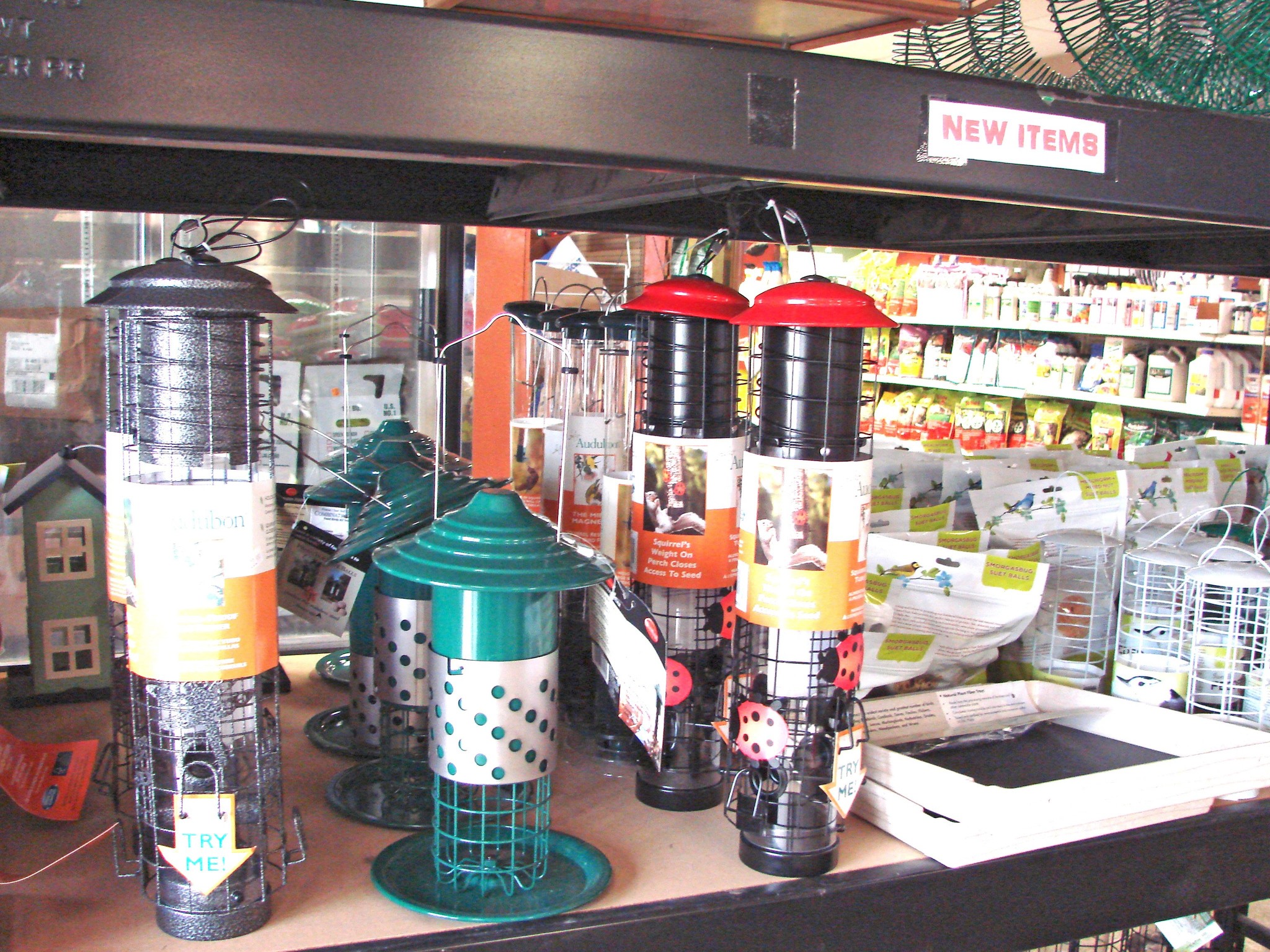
[308,654,610,924]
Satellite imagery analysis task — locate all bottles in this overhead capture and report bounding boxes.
[967,269,1231,336]
[1231,303,1257,334]
[1118,340,1262,410]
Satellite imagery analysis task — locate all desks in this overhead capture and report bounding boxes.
[0,650,1270,952]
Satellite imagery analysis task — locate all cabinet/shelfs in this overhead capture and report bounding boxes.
[862,315,1270,452]
